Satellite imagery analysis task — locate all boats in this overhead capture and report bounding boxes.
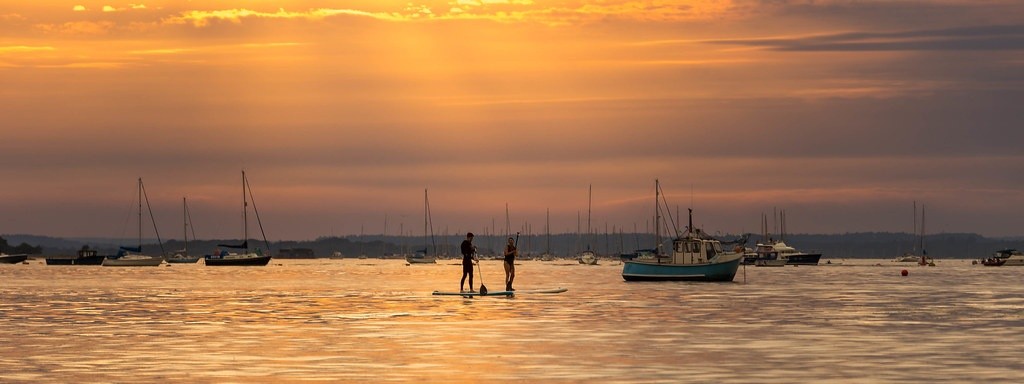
[984,258,1008,266]
[44,244,106,266]
[996,246,1024,266]
[618,178,753,283]
[741,205,825,265]
[754,241,790,267]
[617,247,673,264]
[0,252,29,266]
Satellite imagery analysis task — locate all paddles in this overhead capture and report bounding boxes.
[475,248,487,295]
[515,232,519,249]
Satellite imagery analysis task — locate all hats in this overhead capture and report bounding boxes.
[508,237,515,242]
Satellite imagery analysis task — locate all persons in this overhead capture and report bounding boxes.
[460,232,479,293]
[503,237,518,291]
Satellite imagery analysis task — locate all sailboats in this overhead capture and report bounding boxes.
[405,188,438,264]
[202,168,274,267]
[326,199,682,265]
[164,197,202,264]
[890,201,935,267]
[570,184,602,266]
[101,177,169,267]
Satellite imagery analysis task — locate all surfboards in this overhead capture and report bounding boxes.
[432,288,568,295]
